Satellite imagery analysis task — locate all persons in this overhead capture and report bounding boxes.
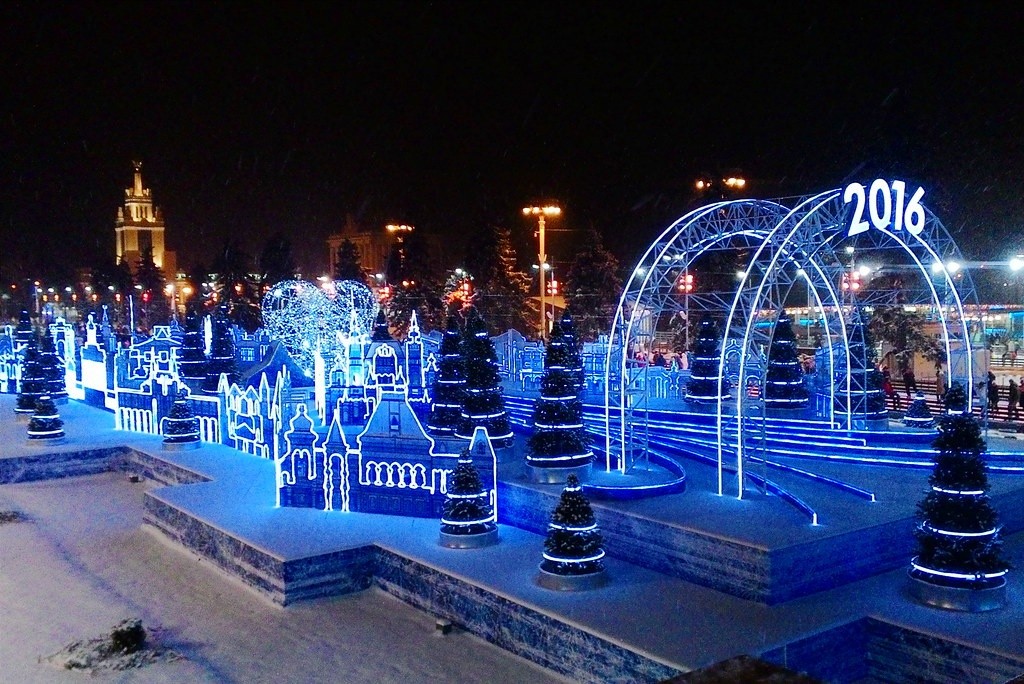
[637,351,683,369]
[883,352,1024,420]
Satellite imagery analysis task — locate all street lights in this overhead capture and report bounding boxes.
[64,286,76,307]
[83,285,96,308]
[530,260,558,322]
[108,285,120,322]
[521,195,561,339]
[384,219,415,286]
[134,285,148,328]
[664,253,688,354]
